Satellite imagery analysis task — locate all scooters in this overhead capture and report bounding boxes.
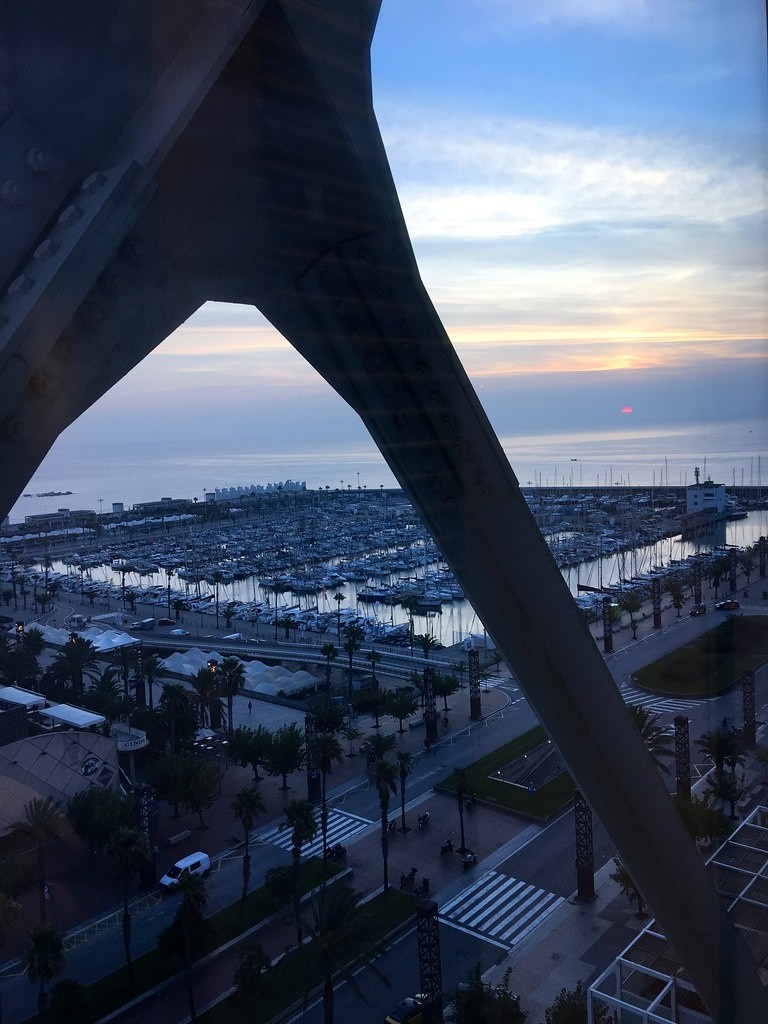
[440,832,455,855]
[400,867,417,891]
[414,876,431,897]
[387,818,396,833]
[417,811,430,830]
[464,791,477,811]
[326,843,346,860]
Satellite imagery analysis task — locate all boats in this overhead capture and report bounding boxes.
[0,483,768,652]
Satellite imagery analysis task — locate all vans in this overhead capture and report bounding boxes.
[159,851,212,894]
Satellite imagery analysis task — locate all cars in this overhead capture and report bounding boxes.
[385,992,431,1024]
